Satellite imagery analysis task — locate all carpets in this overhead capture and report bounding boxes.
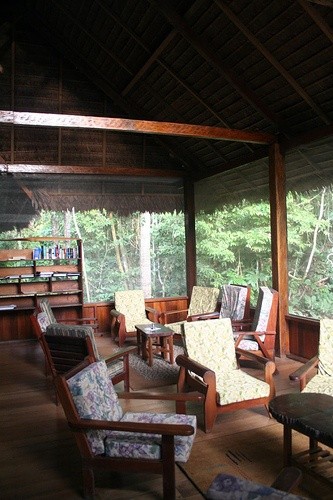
[113,345,186,382]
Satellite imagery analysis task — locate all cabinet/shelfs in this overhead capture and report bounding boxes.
[0,237,85,343]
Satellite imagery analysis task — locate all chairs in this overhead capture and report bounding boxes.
[30,284,333,500]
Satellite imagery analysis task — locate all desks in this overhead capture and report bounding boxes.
[269,393,333,478]
[135,324,175,368]
